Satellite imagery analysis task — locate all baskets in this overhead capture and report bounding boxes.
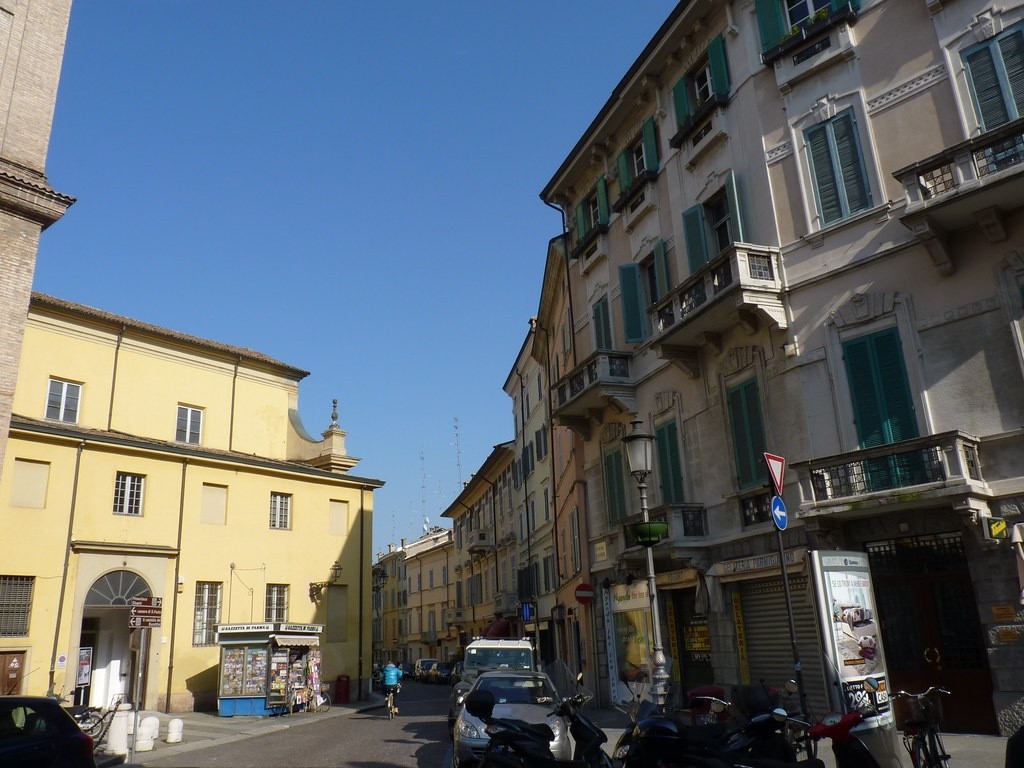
[321,684,329,692]
[908,696,943,728]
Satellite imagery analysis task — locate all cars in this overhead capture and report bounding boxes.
[0,694,98,768]
[450,661,464,688]
[399,663,415,681]
[420,662,434,684]
[430,661,453,685]
[454,669,572,768]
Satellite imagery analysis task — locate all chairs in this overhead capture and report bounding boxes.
[21,711,37,734]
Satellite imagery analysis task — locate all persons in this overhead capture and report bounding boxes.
[632,671,647,683]
[396,661,411,671]
[382,662,403,713]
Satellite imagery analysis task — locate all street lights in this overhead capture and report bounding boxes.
[620,418,670,713]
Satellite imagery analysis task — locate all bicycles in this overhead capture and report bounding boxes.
[888,686,952,768]
[385,688,399,721]
[315,677,331,713]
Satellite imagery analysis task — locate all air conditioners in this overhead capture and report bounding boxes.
[467,528,489,548]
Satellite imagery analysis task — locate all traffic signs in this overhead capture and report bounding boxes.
[128,615,161,628]
[131,595,162,606]
[129,605,162,618]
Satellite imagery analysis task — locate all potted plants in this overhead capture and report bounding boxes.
[675,97,709,133]
[617,175,643,200]
[779,7,830,51]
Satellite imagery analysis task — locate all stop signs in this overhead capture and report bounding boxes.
[575,584,594,605]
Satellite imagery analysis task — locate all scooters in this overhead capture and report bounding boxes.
[773,677,890,768]
[536,659,825,767]
[44,671,104,738]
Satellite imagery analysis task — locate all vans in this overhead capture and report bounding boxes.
[415,659,438,683]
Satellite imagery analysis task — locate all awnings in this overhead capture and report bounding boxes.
[274,635,320,646]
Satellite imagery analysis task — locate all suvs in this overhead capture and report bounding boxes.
[447,636,543,743]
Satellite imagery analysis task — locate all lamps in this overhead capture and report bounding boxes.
[309,560,344,602]
[622,573,634,585]
[602,577,610,588]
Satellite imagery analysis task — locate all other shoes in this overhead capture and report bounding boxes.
[385,698,388,703]
[394,708,398,713]
